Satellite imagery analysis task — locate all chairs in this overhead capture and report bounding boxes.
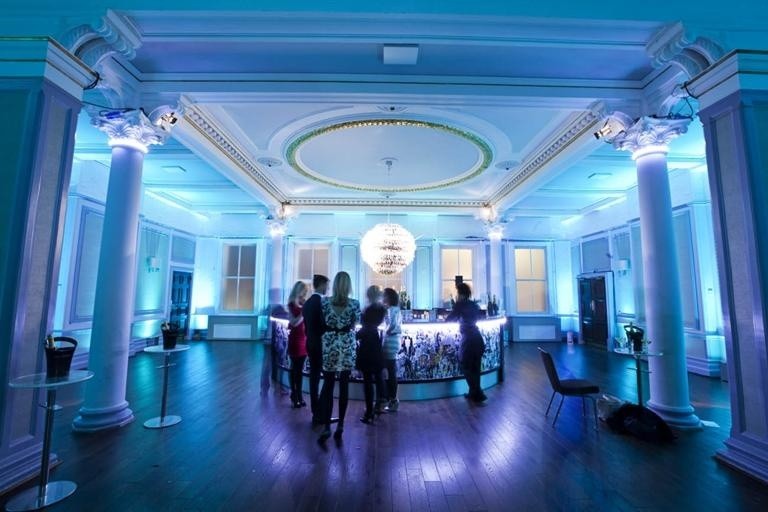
[536,346,601,433]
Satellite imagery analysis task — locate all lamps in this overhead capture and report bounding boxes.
[358,156,417,277]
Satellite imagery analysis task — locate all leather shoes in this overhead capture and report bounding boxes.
[312,417,343,443]
[275,388,288,395]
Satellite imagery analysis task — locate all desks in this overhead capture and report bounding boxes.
[3,369,96,512]
[142,343,192,430]
[612,348,665,406]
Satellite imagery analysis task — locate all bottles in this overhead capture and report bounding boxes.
[164,320,169,332]
[413,310,444,321]
[628,322,634,334]
[486,294,499,316]
[46,334,56,351]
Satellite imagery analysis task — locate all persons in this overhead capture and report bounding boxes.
[258,284,289,397]
[286,280,308,411]
[301,273,341,430]
[444,283,488,404]
[356,284,387,424]
[380,288,403,412]
[315,270,361,448]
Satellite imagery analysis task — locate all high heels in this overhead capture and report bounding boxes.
[374,407,380,419]
[383,397,399,411]
[360,412,375,422]
[299,396,305,406]
[290,393,301,408]
